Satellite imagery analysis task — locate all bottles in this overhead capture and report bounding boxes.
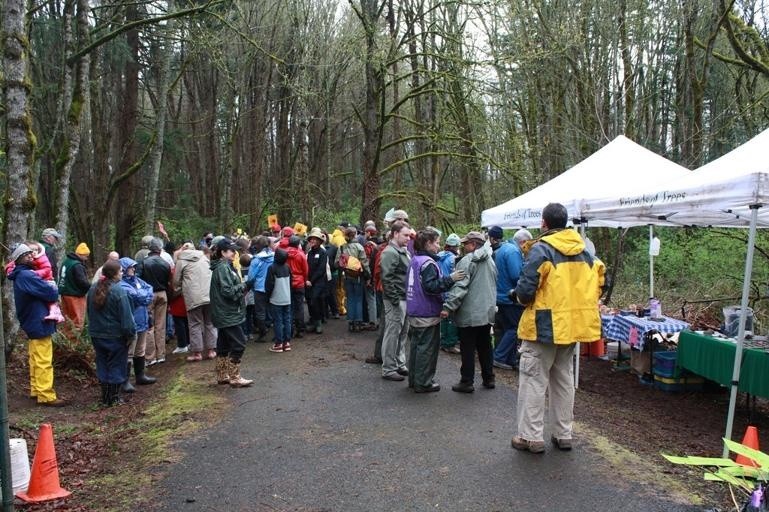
[649,237,660,255]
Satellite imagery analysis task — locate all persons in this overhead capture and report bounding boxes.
[366,207,416,363]
[441,231,496,392]
[366,226,377,242]
[495,229,533,370]
[324,231,340,320]
[381,220,411,381]
[511,203,607,453]
[93,252,119,285]
[246,238,278,342]
[284,238,308,338]
[135,237,171,367]
[341,220,351,227]
[137,235,176,273]
[358,220,375,245]
[305,234,328,333]
[267,248,293,352]
[364,240,388,331]
[210,240,255,386]
[86,261,137,408]
[114,257,156,391]
[212,222,321,239]
[200,233,213,245]
[374,230,390,320]
[336,228,372,331]
[6,243,69,407]
[437,233,462,354]
[173,243,217,361]
[58,244,91,348]
[37,227,60,279]
[8,245,66,324]
[488,227,506,261]
[407,227,466,393]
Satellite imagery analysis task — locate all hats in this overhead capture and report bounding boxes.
[40,228,62,240]
[208,235,225,250]
[445,234,462,247]
[382,209,409,222]
[460,232,487,245]
[8,241,33,262]
[271,224,282,234]
[218,238,243,252]
[281,225,294,236]
[307,231,329,242]
[488,226,504,240]
[363,226,378,233]
[74,242,91,255]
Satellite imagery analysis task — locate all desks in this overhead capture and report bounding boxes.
[601,309,622,358]
[615,311,691,385]
[680,329,769,408]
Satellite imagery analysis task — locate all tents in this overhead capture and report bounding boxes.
[481,126,769,458]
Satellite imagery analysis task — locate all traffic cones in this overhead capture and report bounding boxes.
[733,427,764,471]
[16,423,73,503]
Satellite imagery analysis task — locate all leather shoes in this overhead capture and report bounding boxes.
[382,371,405,382]
[398,366,409,376]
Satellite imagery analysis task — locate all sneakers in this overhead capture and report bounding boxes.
[451,383,475,393]
[408,380,414,387]
[356,325,364,333]
[37,398,65,407]
[480,379,496,388]
[550,434,573,450]
[208,350,217,360]
[186,352,202,361]
[413,382,441,394]
[443,346,460,354]
[29,394,38,399]
[144,360,157,367]
[493,360,512,371]
[172,346,188,354]
[511,435,544,453]
[284,340,292,351]
[158,357,166,363]
[269,343,283,353]
[333,312,341,319]
[347,324,354,333]
[366,356,383,364]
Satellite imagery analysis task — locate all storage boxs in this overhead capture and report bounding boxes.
[653,351,705,394]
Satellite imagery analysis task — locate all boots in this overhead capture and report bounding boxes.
[101,384,108,404]
[133,356,157,385]
[106,383,124,406]
[255,319,267,343]
[121,362,136,393]
[315,320,323,333]
[214,357,230,385]
[228,356,253,388]
